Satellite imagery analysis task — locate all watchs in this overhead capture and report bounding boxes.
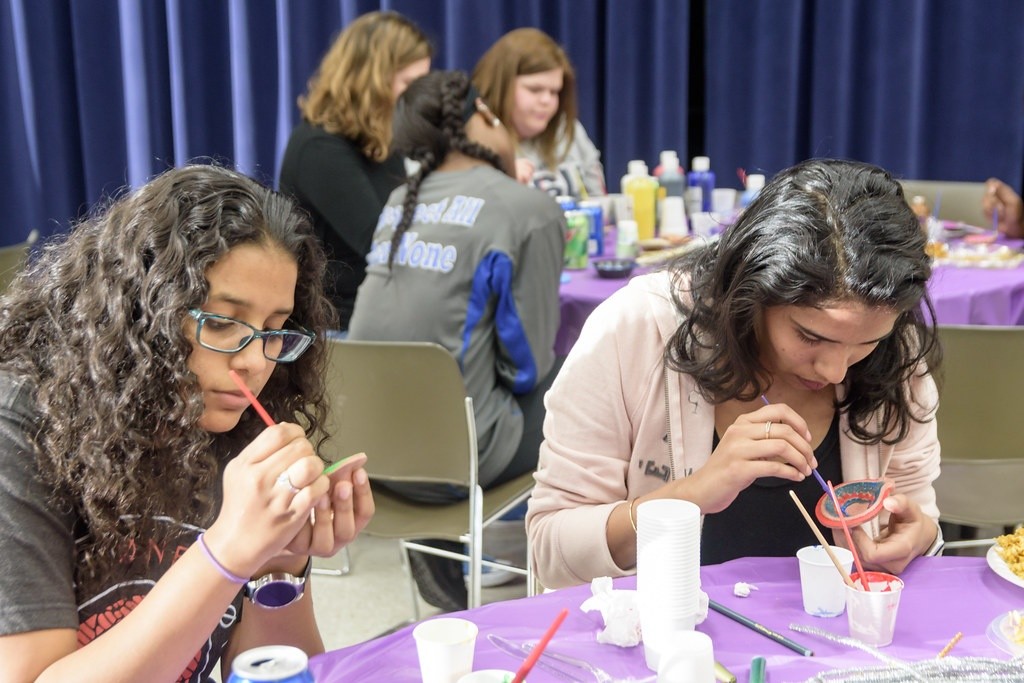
[241,556,312,610]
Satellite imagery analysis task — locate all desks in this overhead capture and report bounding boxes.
[308,545,1023,683]
[547,215,1024,354]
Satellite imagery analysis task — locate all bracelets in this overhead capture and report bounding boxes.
[629,497,638,535]
[197,533,249,586]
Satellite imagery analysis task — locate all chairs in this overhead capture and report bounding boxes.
[925,323,1024,542]
[295,337,537,610]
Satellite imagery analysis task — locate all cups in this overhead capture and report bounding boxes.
[456,670,527,683]
[635,497,717,683]
[842,572,905,647]
[796,545,854,616]
[413,617,479,683]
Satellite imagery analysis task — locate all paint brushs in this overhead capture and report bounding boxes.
[992,207,998,237]
[786,481,869,593]
[510,610,569,683]
[230,371,275,427]
[710,600,814,658]
[762,395,846,515]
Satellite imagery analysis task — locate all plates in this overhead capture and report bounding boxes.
[986,543,1024,588]
[986,610,1024,656]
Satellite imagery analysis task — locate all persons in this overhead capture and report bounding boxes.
[280,10,608,617]
[524,158,947,592]
[981,176,1024,240]
[0,163,374,683]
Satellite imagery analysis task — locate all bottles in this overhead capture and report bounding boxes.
[616,149,764,259]
[911,196,926,234]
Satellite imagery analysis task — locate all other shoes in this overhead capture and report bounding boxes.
[408,535,470,612]
[458,542,517,587]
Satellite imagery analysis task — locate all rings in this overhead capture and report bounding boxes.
[280,470,301,495]
[766,421,772,440]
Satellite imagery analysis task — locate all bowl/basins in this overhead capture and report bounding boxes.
[594,259,637,278]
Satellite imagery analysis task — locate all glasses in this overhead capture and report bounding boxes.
[189,308,318,364]
